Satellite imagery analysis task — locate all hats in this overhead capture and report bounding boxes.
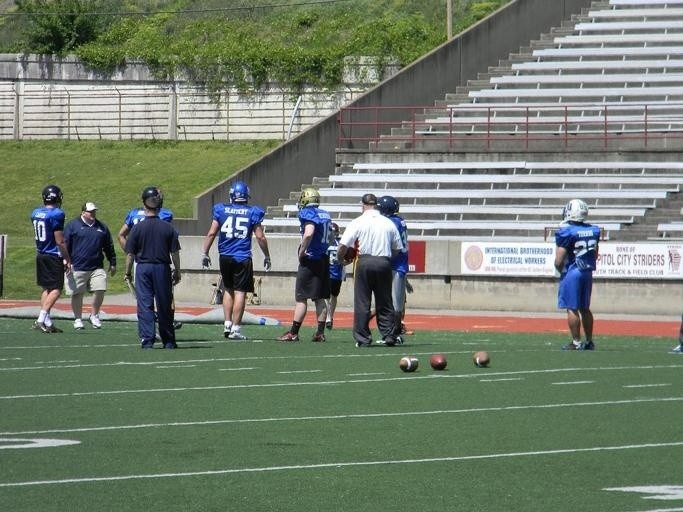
[362,194,378,206]
[82,203,99,212]
[144,196,160,209]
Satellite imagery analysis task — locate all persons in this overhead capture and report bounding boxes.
[63,202,117,332]
[200,181,272,341]
[667,314,683,354]
[30,184,72,334]
[325,222,347,330]
[117,185,174,254]
[336,193,404,347]
[276,187,332,342]
[124,196,182,351]
[376,195,410,337]
[552,198,601,352]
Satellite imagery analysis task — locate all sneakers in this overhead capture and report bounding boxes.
[32,320,63,333]
[400,328,406,334]
[668,345,683,354]
[73,319,85,329]
[561,340,596,350]
[356,343,370,348]
[142,343,177,349]
[174,321,182,329]
[90,313,102,328]
[376,336,403,346]
[223,326,247,340]
[326,322,332,328]
[277,332,299,341]
[312,334,325,343]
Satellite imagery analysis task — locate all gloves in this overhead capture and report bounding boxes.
[64,259,71,276]
[172,270,181,285]
[202,254,211,268]
[264,256,272,272]
[108,266,116,276]
[124,273,132,282]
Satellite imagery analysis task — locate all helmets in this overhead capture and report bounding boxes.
[142,188,158,200]
[229,181,250,203]
[330,224,339,233]
[299,188,320,208]
[42,185,63,205]
[377,196,399,213]
[562,200,589,222]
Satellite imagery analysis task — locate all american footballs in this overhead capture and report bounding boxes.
[473,352,488,367]
[431,354,447,371]
[400,357,419,372]
